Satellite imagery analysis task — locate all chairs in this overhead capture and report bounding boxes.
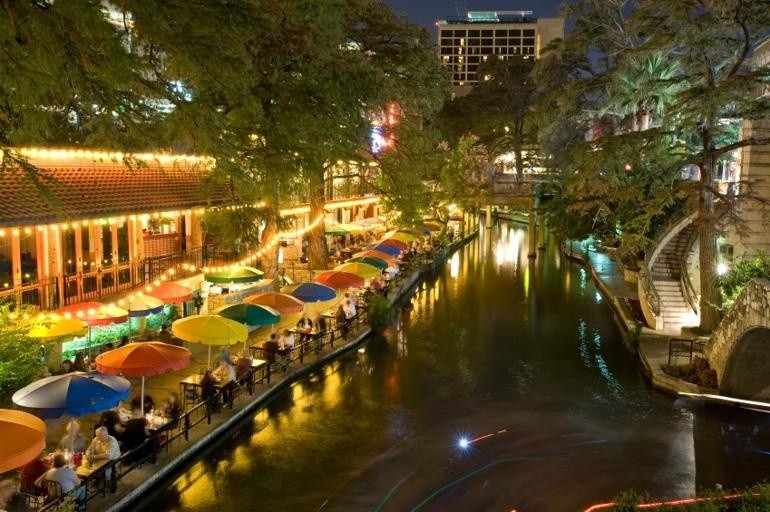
[0,283,391,512]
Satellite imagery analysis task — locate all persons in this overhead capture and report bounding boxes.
[21,449,49,506]
[46,454,87,511]
[85,425,122,493]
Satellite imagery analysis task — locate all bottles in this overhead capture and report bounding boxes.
[74,448,82,466]
